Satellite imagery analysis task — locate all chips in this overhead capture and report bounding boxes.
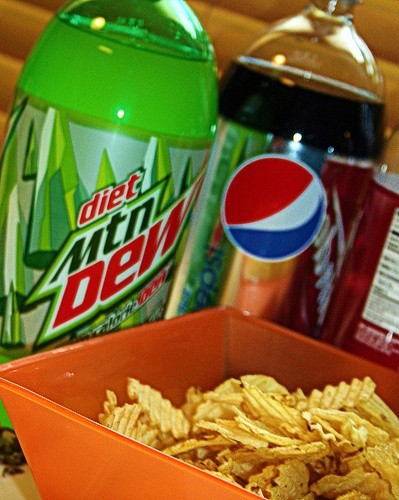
[95,374,398,500]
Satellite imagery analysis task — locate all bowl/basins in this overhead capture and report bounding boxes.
[0,305,399,500]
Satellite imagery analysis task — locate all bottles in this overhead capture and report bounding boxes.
[320,125,399,373]
[163,0,386,344]
[0,1,220,465]
